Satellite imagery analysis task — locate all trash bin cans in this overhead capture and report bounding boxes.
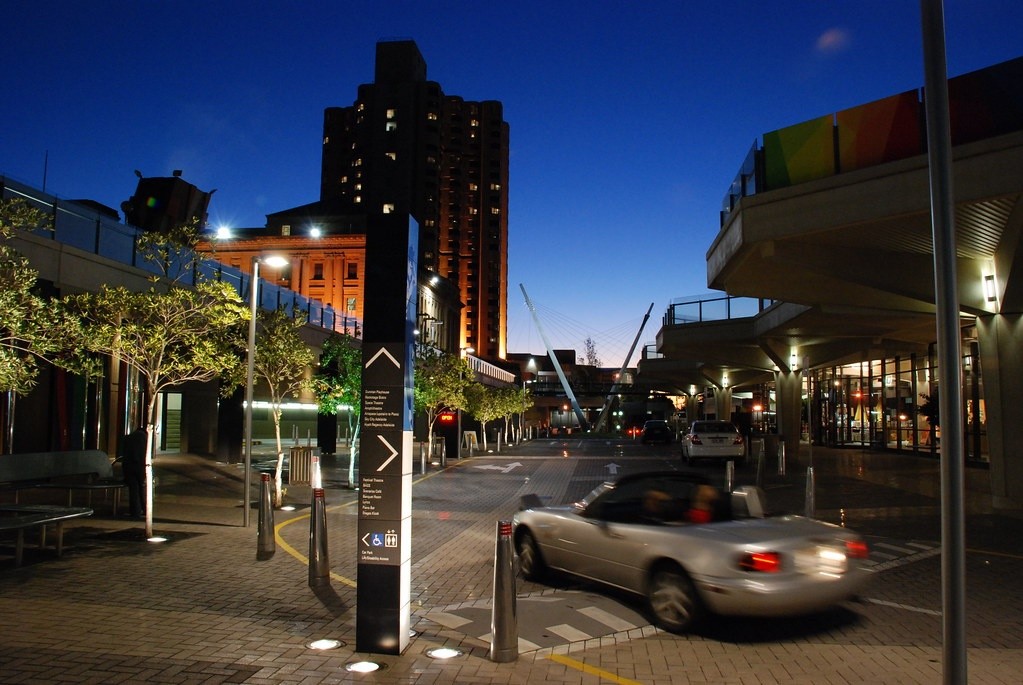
[763,434,779,458]
[432,437,445,456]
[289,446,321,484]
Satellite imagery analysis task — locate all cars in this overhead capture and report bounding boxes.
[679,421,744,467]
[513,472,876,634]
[640,421,673,444]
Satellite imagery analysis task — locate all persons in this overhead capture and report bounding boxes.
[322,302,334,329]
[123,414,150,520]
[683,484,720,524]
[629,471,677,523]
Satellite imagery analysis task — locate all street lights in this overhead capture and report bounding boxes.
[243,254,289,526]
